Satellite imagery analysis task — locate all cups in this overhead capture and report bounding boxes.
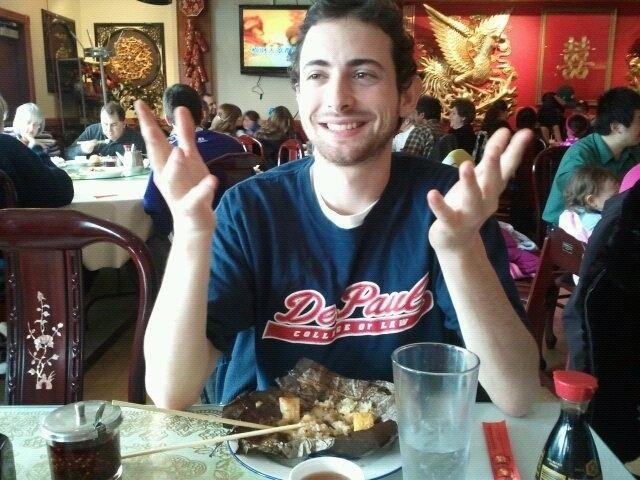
[391,342,480,480]
[75,156,86,162]
[288,456,365,480]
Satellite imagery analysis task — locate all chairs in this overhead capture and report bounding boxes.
[277,139,304,169]
[532,146,581,348]
[524,227,587,386]
[1,209,159,404]
[205,152,270,209]
[472,133,485,164]
[237,134,265,155]
[1,170,17,210]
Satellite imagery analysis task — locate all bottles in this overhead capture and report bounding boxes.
[123,144,131,154]
[39,399,124,480]
[535,369,603,480]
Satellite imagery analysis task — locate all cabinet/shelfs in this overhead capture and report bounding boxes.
[56,57,97,132]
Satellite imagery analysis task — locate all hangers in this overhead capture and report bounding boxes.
[252,75,264,96]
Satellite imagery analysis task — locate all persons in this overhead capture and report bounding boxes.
[1,98,98,285]
[392,87,639,288]
[126,83,245,275]
[64,101,146,161]
[200,93,304,175]
[6,102,60,158]
[134,0,539,418]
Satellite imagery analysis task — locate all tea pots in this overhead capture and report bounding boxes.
[115,144,150,173]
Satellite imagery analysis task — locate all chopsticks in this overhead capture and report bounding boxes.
[77,140,105,144]
[19,139,56,143]
[112,400,306,459]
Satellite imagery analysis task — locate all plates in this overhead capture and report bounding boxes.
[80,168,123,179]
[225,430,402,480]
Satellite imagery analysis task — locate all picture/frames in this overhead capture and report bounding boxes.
[94,22,166,110]
[41,8,78,93]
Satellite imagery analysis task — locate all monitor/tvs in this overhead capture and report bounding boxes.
[239,5,313,76]
